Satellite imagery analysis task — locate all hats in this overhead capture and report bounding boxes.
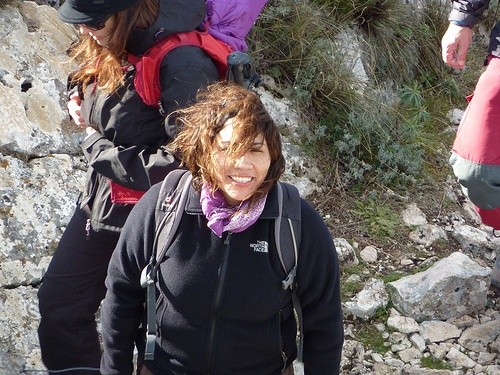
[57,0,116,24]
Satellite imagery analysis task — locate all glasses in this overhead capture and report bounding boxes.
[74,15,113,31]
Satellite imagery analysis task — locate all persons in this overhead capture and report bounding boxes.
[97,83,343,375]
[37,0,218,375]
[441,0,500,287]
